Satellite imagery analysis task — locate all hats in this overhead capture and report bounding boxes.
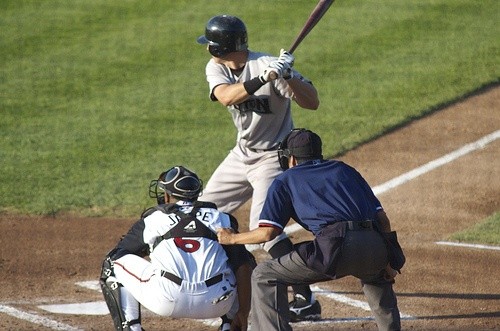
[287,129,321,157]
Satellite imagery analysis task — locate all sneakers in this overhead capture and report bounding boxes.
[288,300,321,323]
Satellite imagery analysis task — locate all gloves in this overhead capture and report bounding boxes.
[259,60,287,84]
[279,48,295,80]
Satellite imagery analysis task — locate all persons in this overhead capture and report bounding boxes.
[98,166,258,331]
[216,127,406,331]
[196,13,323,324]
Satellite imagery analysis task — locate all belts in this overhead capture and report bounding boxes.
[161,271,222,289]
[346,220,376,231]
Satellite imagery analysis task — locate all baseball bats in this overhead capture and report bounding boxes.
[269,0,334,79]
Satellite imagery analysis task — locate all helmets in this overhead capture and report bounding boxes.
[150,165,203,200]
[196,14,248,57]
